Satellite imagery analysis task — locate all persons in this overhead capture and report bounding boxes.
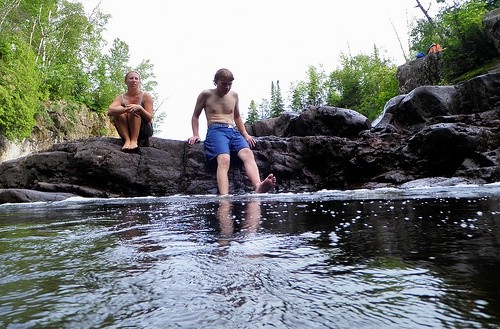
[187,68,276,195]
[108,71,154,149]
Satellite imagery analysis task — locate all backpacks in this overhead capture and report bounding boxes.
[427,43,442,55]
